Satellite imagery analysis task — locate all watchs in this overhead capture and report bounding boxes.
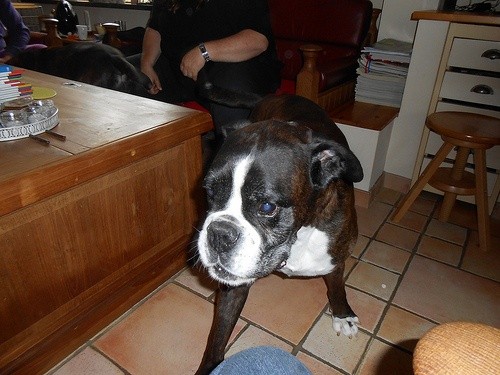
[199,43,211,62]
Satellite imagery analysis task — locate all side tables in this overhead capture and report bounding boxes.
[327,100,401,192]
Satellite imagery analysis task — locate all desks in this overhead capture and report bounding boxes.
[0,61,211,375]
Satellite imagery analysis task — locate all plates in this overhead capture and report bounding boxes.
[31,87,57,99]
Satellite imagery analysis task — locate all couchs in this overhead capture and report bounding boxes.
[102,0,383,130]
[27,18,64,50]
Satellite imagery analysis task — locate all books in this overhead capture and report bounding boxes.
[0,64,34,103]
[354,39,414,108]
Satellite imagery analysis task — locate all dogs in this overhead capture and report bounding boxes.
[5,43,151,95]
[194,92,365,375]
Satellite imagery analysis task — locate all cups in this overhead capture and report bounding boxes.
[0,110,26,128]
[76,25,87,40]
[28,99,55,118]
[21,106,42,124]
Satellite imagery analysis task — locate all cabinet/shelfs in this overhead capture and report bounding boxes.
[384,10,500,216]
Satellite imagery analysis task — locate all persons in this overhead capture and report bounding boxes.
[0,0,30,66]
[124,0,282,152]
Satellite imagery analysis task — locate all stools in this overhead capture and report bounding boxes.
[391,111,500,252]
